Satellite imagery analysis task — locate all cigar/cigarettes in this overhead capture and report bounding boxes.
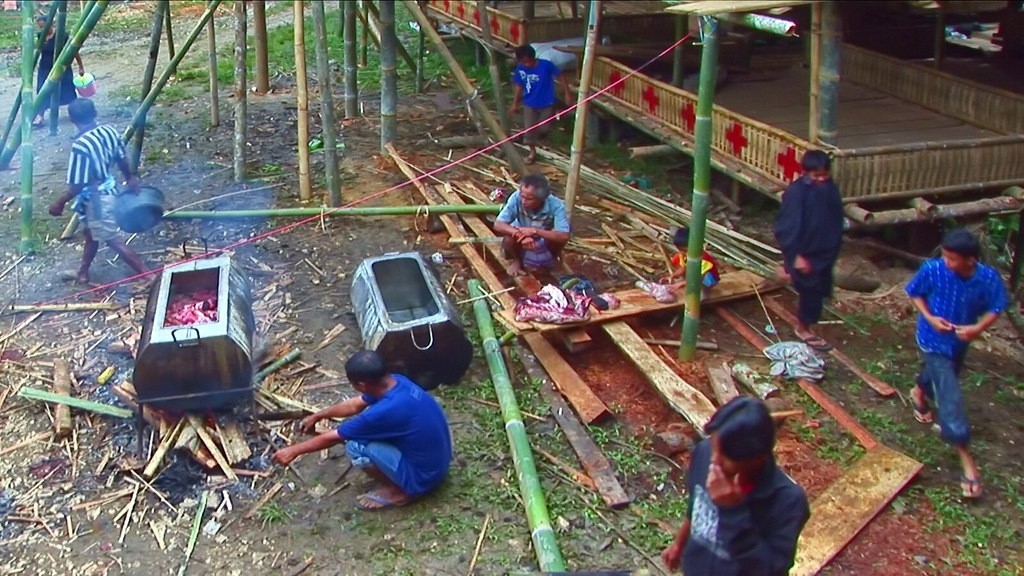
[710,466,719,475]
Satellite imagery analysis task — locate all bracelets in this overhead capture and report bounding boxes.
[61,188,74,199]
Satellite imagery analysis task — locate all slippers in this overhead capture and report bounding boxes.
[909,392,933,423]
[525,152,537,165]
[798,330,834,350]
[355,486,412,511]
[959,472,984,499]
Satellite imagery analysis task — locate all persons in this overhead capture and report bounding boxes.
[659,226,721,301]
[49,98,157,288]
[906,226,1008,497]
[660,396,810,576]
[271,349,451,510]
[775,149,844,350]
[32,16,85,128]
[508,44,575,164]
[492,174,571,276]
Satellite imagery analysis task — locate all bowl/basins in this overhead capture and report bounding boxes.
[113,185,166,232]
